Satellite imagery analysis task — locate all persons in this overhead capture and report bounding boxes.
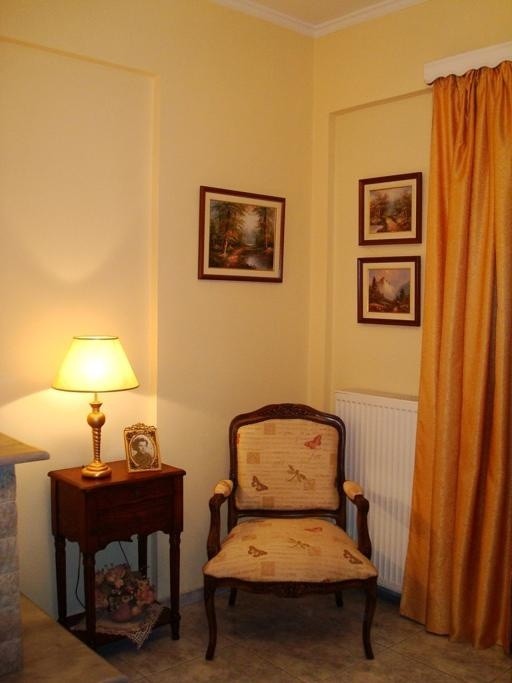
[131,437,153,466]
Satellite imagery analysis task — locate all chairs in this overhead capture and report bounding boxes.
[201,403,377,660]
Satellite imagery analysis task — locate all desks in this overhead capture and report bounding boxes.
[0,430,50,682]
[48,458,186,651]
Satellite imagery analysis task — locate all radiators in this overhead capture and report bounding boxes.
[333,390,419,595]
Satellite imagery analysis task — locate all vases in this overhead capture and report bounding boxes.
[108,603,132,624]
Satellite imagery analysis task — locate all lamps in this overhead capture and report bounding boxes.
[52,335,141,481]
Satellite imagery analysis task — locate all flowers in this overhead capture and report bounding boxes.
[94,563,156,609]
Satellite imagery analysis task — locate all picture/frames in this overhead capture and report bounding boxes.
[358,172,422,245]
[356,256,421,326]
[199,185,286,283]
[123,424,161,473]
